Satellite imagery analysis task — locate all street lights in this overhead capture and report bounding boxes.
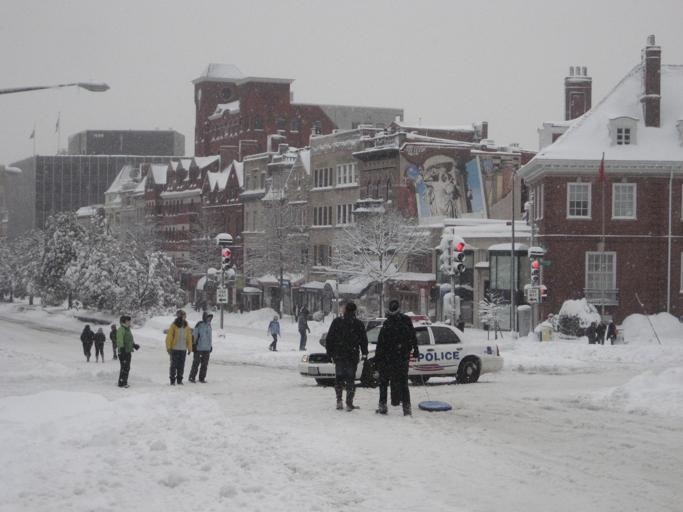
[0,164,22,175]
[0,81,112,94]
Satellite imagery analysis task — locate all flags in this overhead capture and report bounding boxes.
[598,158,609,183]
[29,131,35,140]
[56,118,60,132]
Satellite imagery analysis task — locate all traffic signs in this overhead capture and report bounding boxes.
[215,288,228,304]
[525,288,539,304]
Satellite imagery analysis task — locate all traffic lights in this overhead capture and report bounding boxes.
[530,259,539,284]
[221,247,232,273]
[539,284,548,303]
[434,239,450,273]
[452,239,465,277]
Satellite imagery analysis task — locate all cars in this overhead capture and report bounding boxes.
[297,313,503,390]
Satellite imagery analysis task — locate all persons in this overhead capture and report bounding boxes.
[326,301,419,416]
[195,297,207,312]
[116,316,140,388]
[239,301,244,314]
[188,311,213,384]
[454,314,465,333]
[266,316,282,351]
[586,319,617,345]
[293,303,310,351]
[80,324,117,363]
[165,310,193,386]
